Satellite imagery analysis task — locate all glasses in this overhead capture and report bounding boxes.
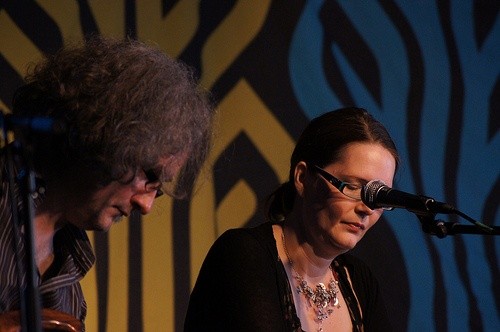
[143,162,164,199]
[306,160,394,211]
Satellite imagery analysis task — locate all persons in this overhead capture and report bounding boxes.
[185,107,399,332]
[0,36,217,332]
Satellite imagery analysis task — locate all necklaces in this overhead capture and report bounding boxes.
[282,222,341,332]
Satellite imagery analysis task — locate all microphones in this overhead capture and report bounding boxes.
[361,178,454,214]
